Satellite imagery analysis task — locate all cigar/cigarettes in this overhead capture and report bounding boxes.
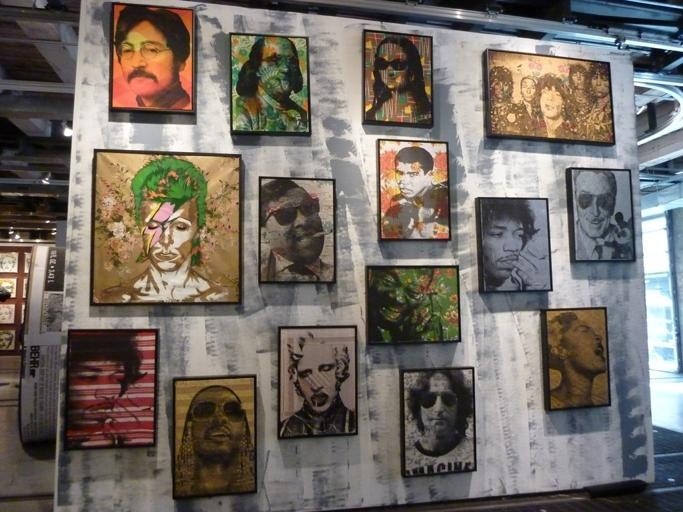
[312,229,333,237]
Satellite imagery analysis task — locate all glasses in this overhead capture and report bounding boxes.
[117,43,171,60]
[375,57,408,70]
[579,194,614,209]
[193,401,245,424]
[418,391,457,409]
[262,198,320,225]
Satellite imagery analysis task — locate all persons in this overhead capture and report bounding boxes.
[277,330,353,433]
[177,385,258,495]
[543,313,606,409]
[235,37,305,130]
[404,370,474,473]
[69,332,149,445]
[114,6,189,108]
[573,169,633,259]
[479,198,546,289]
[0,253,18,350]
[364,37,431,124]
[101,158,238,303]
[487,59,610,139]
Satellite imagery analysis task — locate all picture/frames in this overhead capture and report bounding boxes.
[278,325,358,439]
[89,149,242,306]
[473,197,552,292]
[361,29,433,128]
[566,166,636,261]
[63,329,157,449]
[398,368,477,477]
[481,48,615,146]
[230,31,311,137]
[541,306,612,412]
[171,375,257,500]
[110,2,197,116]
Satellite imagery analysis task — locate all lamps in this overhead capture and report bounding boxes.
[58,120,73,138]
[41,170,53,185]
